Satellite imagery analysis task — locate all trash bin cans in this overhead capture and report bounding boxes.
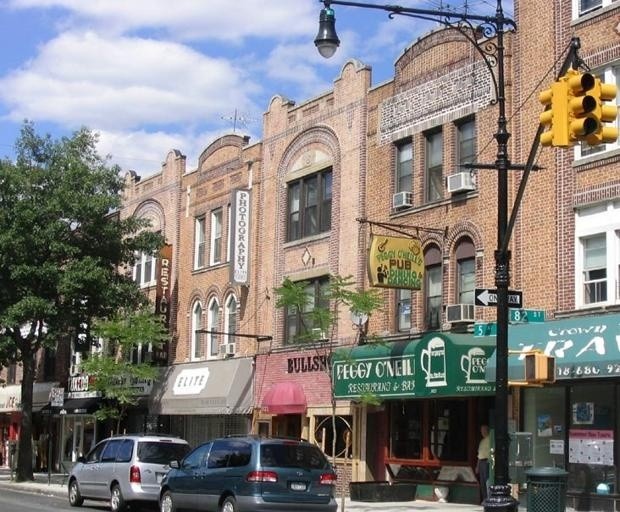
[524,467,570,512]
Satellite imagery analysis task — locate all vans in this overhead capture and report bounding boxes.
[156,431,340,511]
[67,432,192,511]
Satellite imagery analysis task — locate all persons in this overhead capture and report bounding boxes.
[473,424,490,505]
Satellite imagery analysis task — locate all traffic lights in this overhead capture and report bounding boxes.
[541,357,556,383]
[561,71,599,147]
[588,78,619,147]
[538,83,560,147]
[526,354,536,381]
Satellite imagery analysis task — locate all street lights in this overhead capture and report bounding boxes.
[317,1,522,511]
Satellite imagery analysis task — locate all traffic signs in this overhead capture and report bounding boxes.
[474,321,497,337]
[474,289,523,308]
[510,309,545,324]
